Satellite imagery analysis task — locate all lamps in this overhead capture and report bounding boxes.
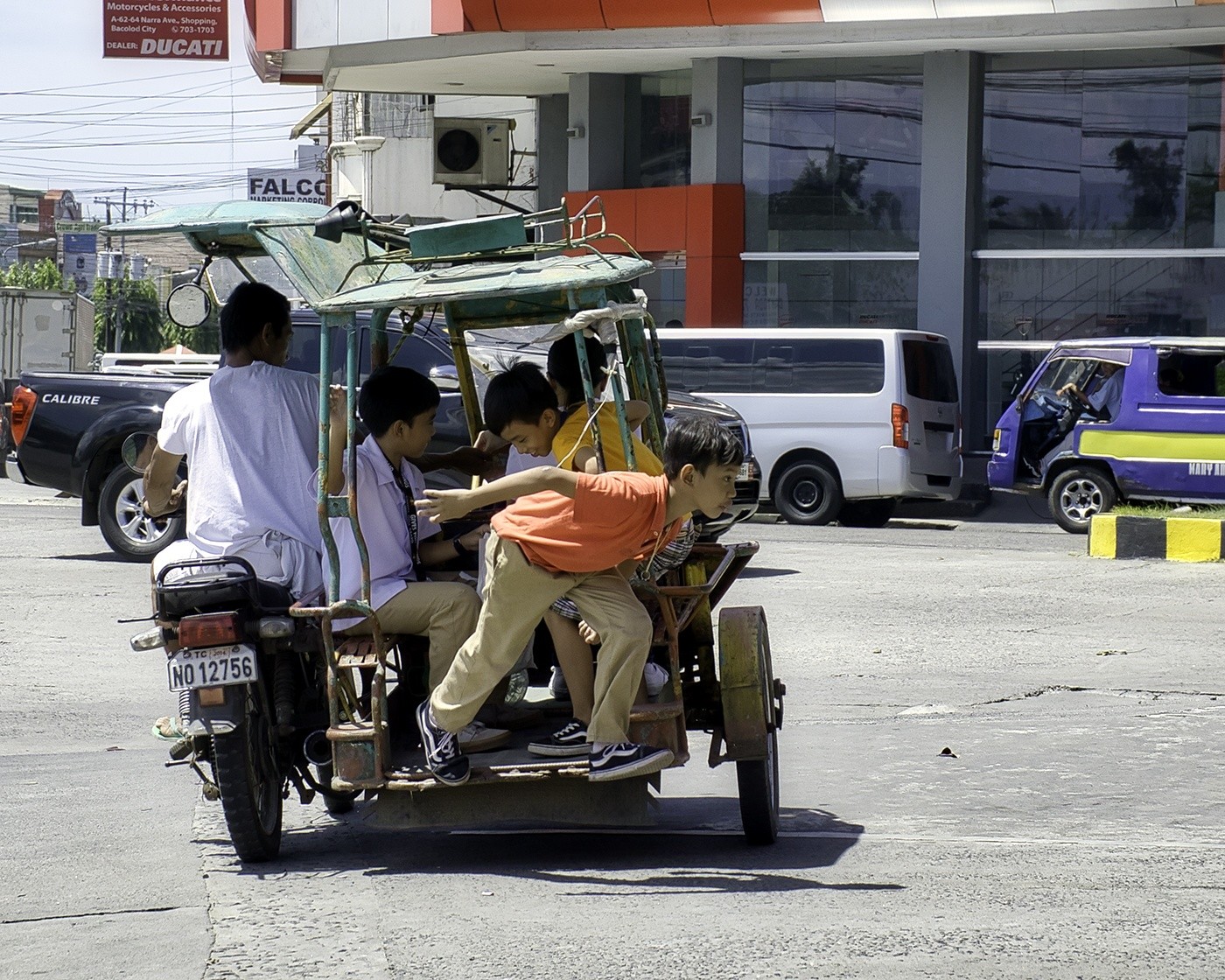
[566,127,585,138]
[692,113,711,126]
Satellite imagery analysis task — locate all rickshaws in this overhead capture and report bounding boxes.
[98,196,787,849]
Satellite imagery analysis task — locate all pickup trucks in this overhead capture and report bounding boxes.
[12,308,500,564]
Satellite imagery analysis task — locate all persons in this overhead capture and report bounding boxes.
[143,281,493,739]
[1023,362,1126,477]
[414,417,745,784]
[472,334,668,696]
[131,433,159,472]
[484,360,690,757]
[322,367,543,752]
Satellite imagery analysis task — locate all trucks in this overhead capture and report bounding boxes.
[0,286,97,381]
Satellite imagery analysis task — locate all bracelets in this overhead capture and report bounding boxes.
[453,531,468,557]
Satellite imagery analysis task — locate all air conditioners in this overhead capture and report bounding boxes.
[432,117,509,185]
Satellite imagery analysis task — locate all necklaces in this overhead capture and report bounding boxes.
[642,523,674,580]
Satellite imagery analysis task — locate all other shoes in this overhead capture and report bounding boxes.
[1023,456,1042,477]
[383,756,432,780]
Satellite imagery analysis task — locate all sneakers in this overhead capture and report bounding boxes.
[589,742,675,782]
[416,698,471,787]
[459,721,510,747]
[548,667,569,695]
[528,716,588,756]
[643,660,670,695]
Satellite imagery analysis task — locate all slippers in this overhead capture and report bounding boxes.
[152,717,184,741]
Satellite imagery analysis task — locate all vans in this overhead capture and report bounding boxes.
[611,327,964,527]
[986,335,1224,535]
